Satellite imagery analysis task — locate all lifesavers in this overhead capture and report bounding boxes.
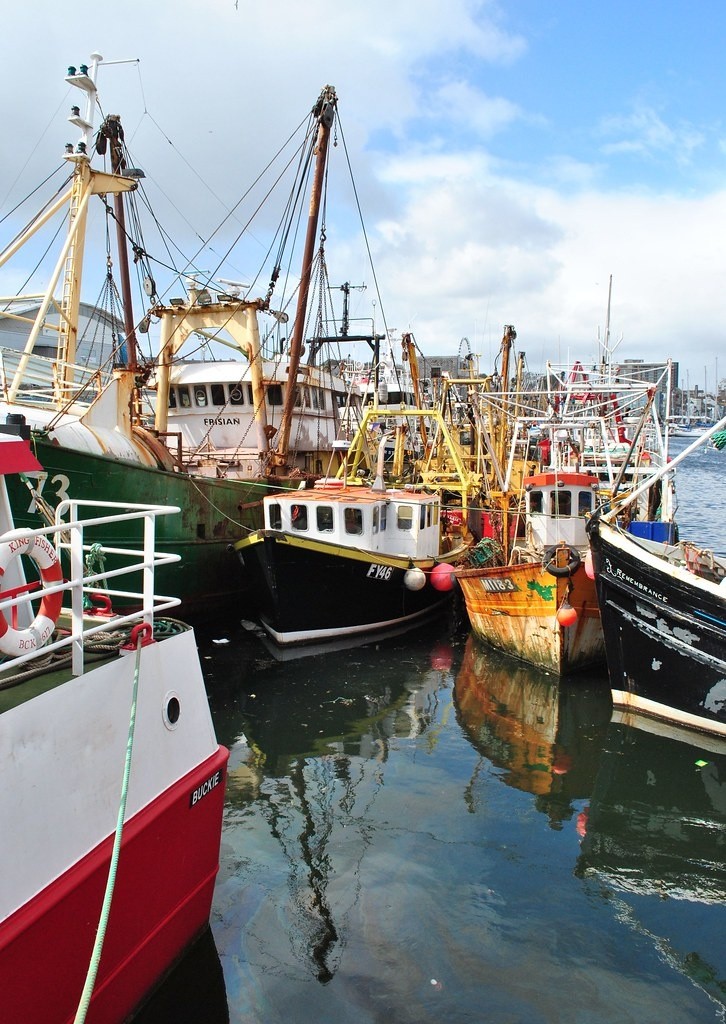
[313,478,345,490]
[542,545,580,577]
[0,528,64,655]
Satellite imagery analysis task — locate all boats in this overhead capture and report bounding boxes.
[0,406,233,1024]
[580,411,725,755]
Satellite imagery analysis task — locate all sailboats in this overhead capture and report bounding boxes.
[0,46,683,683]
